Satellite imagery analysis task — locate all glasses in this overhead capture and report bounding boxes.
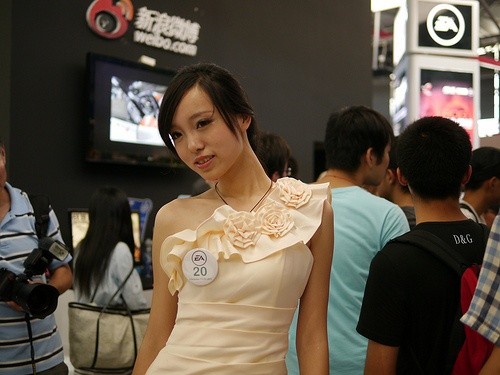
[281,168,291,176]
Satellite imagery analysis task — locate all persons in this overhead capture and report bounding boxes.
[284,104,500,375]
[131,64,334,375]
[0,138,71,374]
[253,131,291,183]
[69,188,147,375]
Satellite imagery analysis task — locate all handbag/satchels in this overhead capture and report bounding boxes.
[68,241,151,369]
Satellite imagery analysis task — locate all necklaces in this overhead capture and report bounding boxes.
[212,180,273,213]
[324,175,356,185]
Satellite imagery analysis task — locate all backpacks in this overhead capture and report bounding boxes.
[393,223,494,374]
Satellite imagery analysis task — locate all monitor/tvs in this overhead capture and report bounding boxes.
[83,53,187,169]
[67,208,142,266]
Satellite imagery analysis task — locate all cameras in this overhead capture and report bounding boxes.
[0,237,71,318]
